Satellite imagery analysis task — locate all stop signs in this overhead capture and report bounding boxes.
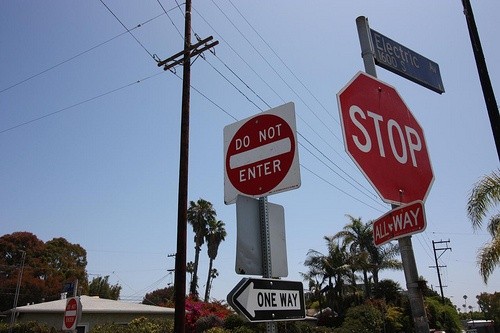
[61,296,80,331]
[220,101,302,203]
[336,69,437,204]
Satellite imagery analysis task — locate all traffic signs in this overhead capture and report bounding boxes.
[369,27,445,94]
